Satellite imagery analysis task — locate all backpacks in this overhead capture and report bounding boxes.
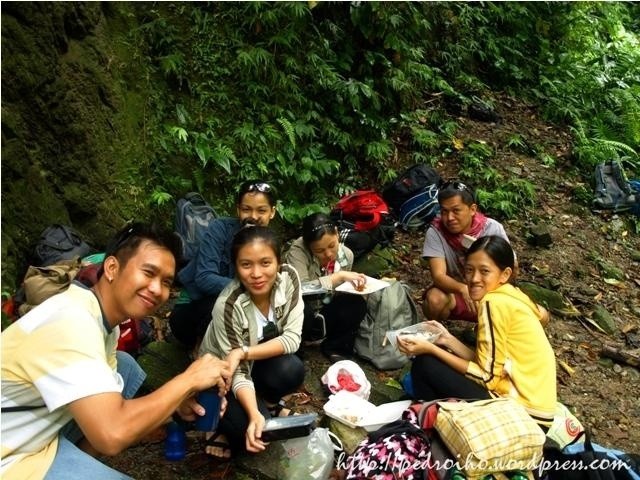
[590,162,634,215]
[390,164,443,223]
[172,191,219,258]
[77,263,143,351]
[344,398,639,479]
[334,190,390,229]
[354,276,420,371]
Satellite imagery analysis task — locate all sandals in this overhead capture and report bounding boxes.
[265,401,304,417]
[204,431,234,461]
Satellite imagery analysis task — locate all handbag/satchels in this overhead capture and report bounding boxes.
[18,223,90,315]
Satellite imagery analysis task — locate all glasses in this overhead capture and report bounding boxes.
[240,182,271,192]
[438,180,473,195]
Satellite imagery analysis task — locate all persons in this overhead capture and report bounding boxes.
[420,174,552,331]
[196,216,308,466]
[164,179,278,352]
[1,219,231,480]
[279,210,369,365]
[396,235,559,438]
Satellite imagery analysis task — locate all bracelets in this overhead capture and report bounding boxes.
[242,345,250,362]
